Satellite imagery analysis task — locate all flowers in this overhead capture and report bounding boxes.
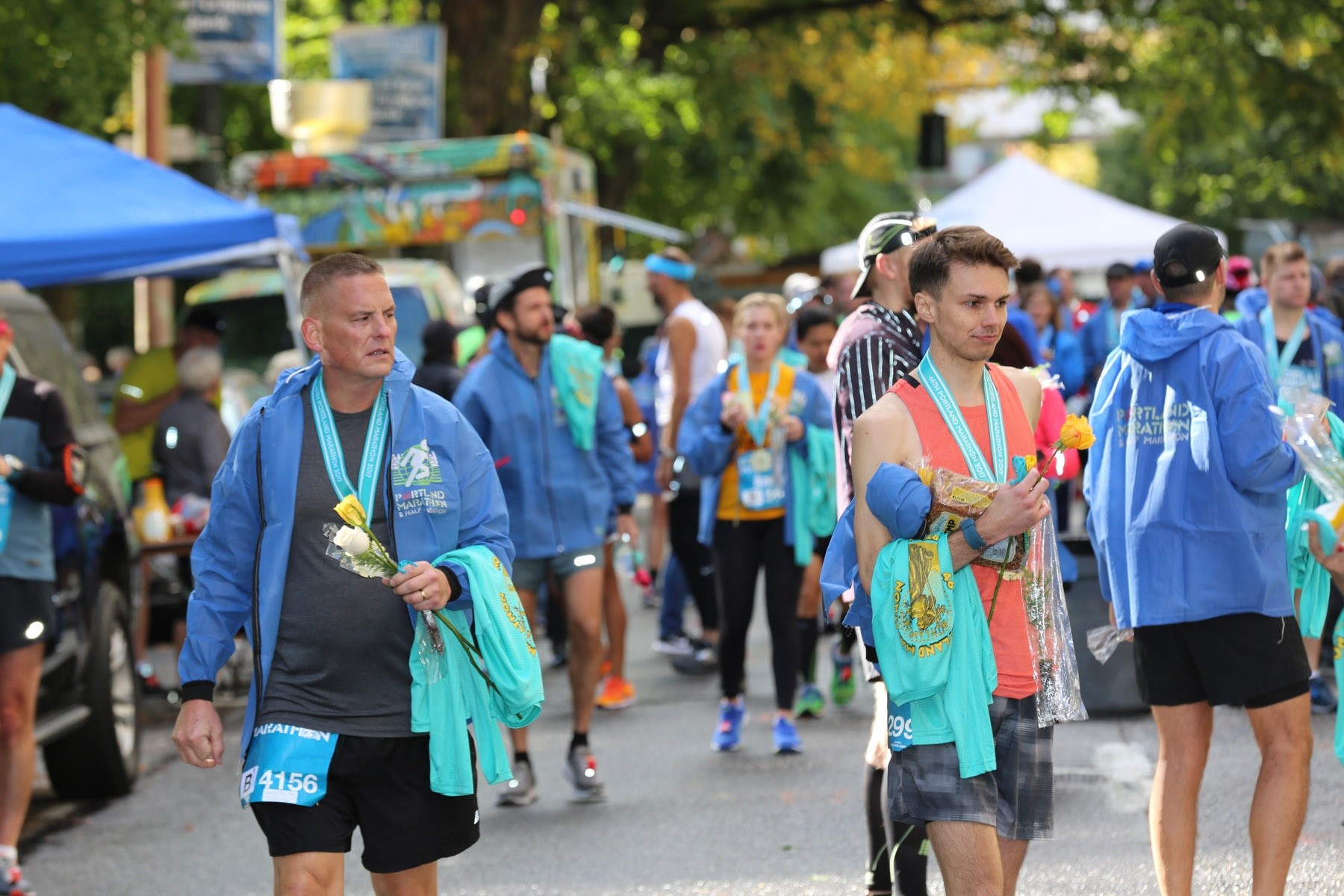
[321,492,525,721]
[987,410,1096,627]
[624,542,651,589]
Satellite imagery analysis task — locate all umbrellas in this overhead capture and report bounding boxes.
[817,154,1228,274]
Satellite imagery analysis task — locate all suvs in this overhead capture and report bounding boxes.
[0,281,149,795]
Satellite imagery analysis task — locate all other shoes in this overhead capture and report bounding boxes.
[712,698,745,753]
[795,685,823,718]
[0,857,34,896]
[674,643,719,672]
[496,762,536,806]
[654,637,693,656]
[772,717,802,755]
[563,745,605,791]
[1309,677,1335,713]
[596,679,636,709]
[831,642,858,703]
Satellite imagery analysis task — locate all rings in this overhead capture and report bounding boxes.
[419,588,427,601]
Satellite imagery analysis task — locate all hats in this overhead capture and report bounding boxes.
[850,211,936,302]
[476,268,554,321]
[1155,222,1222,286]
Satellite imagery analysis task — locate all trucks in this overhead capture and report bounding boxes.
[173,132,688,367]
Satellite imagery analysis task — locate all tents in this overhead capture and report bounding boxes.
[0,100,307,356]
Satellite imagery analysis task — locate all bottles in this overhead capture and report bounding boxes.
[132,478,171,546]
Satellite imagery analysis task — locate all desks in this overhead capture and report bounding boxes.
[133,538,201,669]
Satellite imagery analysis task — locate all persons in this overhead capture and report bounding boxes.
[0,211,1344,896]
[820,225,1051,896]
[170,257,548,894]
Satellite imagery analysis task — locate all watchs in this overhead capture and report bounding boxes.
[628,417,652,440]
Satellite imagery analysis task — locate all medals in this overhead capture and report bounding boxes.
[352,539,391,579]
[750,447,774,473]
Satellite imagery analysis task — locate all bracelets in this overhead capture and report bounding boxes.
[659,448,678,460]
[960,516,988,551]
